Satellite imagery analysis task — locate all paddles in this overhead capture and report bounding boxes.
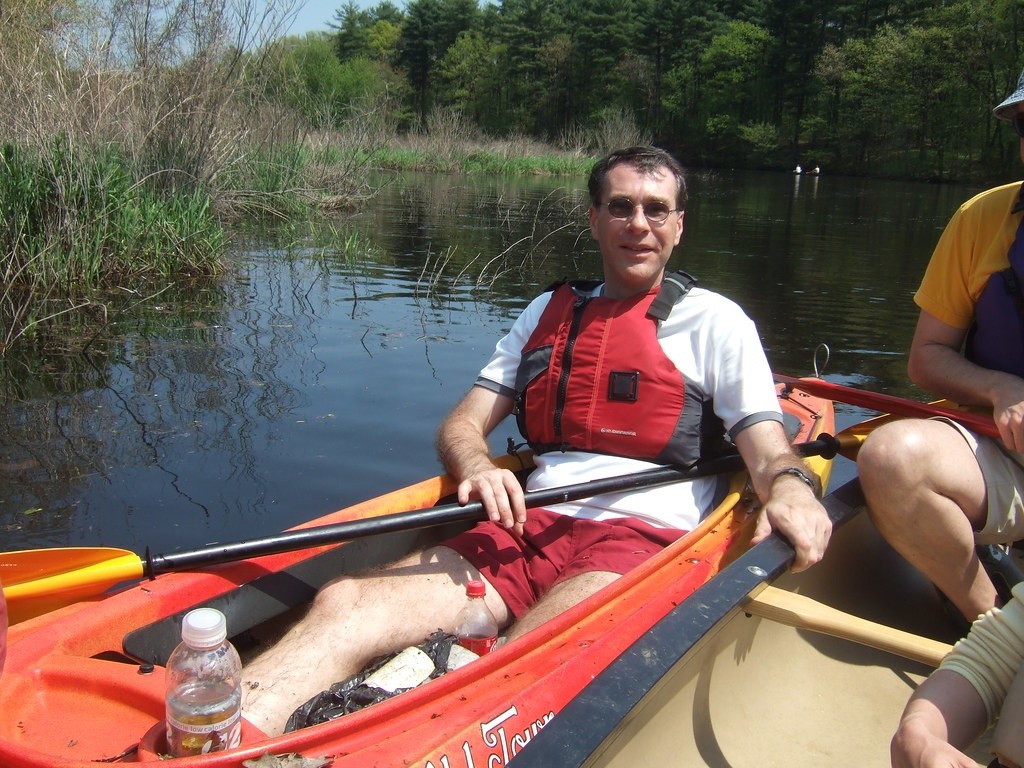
[0,370,1024,630]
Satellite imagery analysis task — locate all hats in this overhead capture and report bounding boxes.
[993,72,1024,122]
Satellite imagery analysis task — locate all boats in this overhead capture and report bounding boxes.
[0,340,837,768]
[498,474,1024,767]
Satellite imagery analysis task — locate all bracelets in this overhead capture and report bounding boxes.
[770,467,816,500]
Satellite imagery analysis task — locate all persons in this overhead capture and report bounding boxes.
[230,146,833,743]
[892,579,1024,768]
[812,165,820,173]
[796,163,802,173]
[857,72,1024,627]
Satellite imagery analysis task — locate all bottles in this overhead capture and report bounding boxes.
[164,607,241,757]
[451,581,498,659]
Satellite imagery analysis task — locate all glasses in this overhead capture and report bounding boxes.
[1013,114,1024,137]
[598,198,682,222]
